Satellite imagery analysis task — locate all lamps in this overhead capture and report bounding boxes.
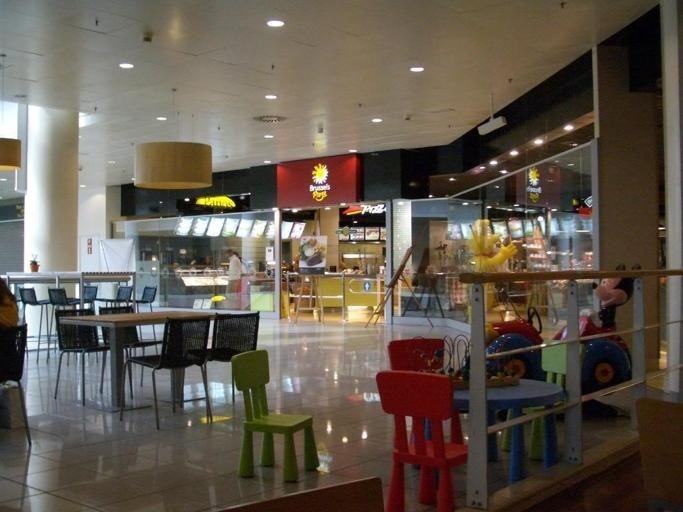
[132,88,213,189]
[0,58,22,169]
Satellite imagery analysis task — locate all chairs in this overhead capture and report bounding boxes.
[0,323,31,446]
[375,370,469,512]
[230,350,319,482]
[118,315,210,430]
[17,284,157,408]
[216,476,384,512]
[499,339,586,461]
[387,338,464,470]
[179,311,260,422]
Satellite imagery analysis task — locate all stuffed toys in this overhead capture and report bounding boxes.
[468,219,518,341]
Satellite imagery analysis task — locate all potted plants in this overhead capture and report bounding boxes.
[30,260,40,272]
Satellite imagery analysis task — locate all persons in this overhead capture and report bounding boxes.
[225,249,251,310]
[0,277,19,378]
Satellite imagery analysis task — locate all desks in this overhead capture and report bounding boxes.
[420,379,565,487]
[0,274,131,363]
[58,310,259,412]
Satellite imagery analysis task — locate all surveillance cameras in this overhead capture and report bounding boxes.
[477,116,508,136]
